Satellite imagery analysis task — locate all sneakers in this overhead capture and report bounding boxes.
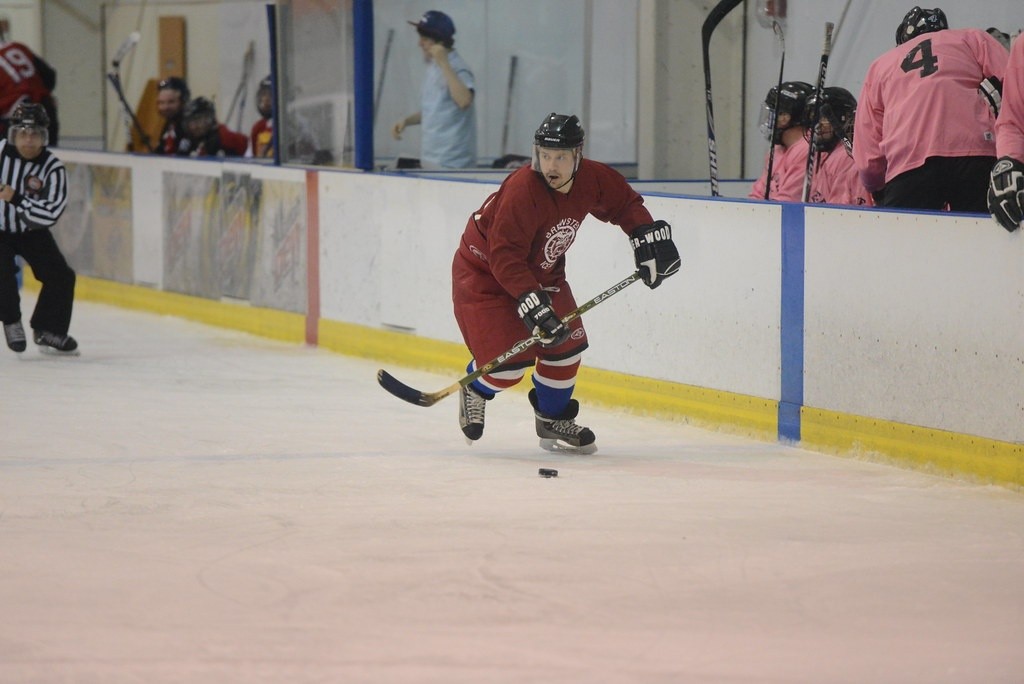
[458,384,495,446]
[32,328,80,356]
[3,320,27,353]
[527,388,598,454]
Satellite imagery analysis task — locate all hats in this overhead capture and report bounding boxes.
[407,10,455,38]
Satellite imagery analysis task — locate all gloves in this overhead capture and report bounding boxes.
[628,220,682,289]
[517,290,574,348]
[987,156,1024,233]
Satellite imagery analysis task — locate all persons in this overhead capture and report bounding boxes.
[746,80,818,202]
[450,112,681,454]
[390,9,480,168]
[801,83,876,206]
[251,73,319,160]
[0,19,60,149]
[150,75,192,155]
[985,29,1024,232]
[0,95,79,356]
[181,95,249,158]
[851,7,1011,215]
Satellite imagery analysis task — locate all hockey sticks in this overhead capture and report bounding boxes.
[374,27,395,120]
[111,32,141,151]
[801,21,834,203]
[107,73,154,152]
[376,269,644,408]
[764,20,787,199]
[501,54,519,155]
[700,0,743,197]
[222,39,255,131]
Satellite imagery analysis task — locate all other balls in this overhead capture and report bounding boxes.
[539,468,558,478]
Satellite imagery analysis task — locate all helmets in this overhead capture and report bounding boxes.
[759,82,817,145]
[4,96,50,127]
[156,77,190,99]
[801,87,857,152]
[896,7,948,47]
[181,97,216,140]
[256,74,290,118]
[532,112,585,149]
